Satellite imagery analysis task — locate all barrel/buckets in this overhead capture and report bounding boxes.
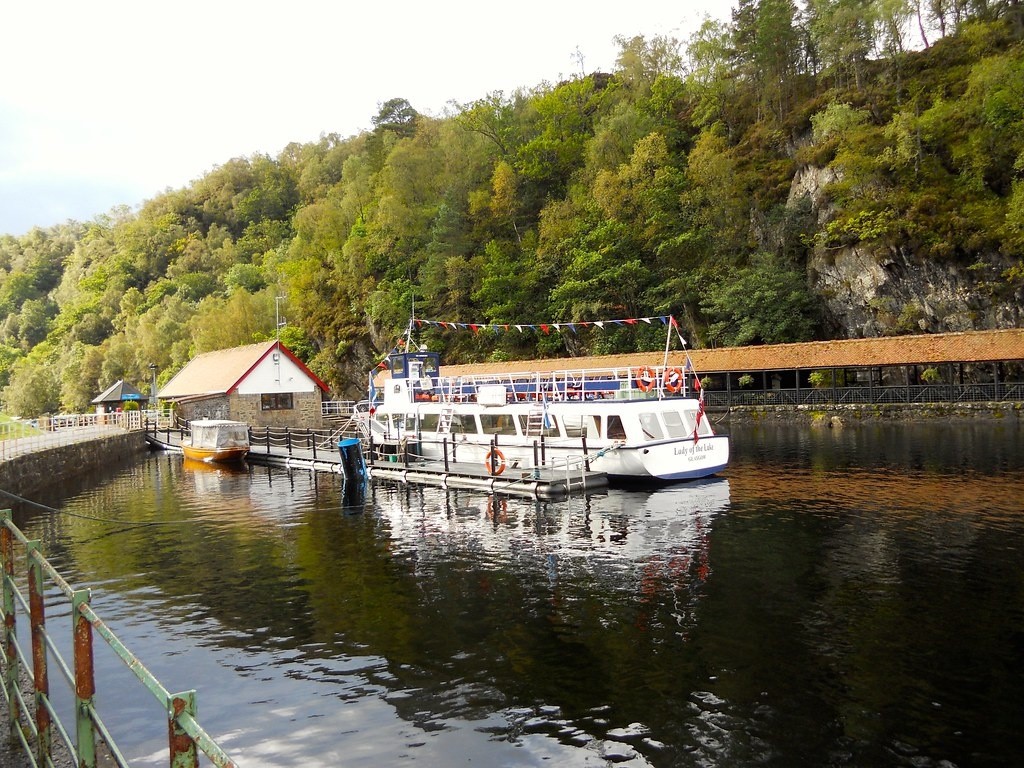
[364,443,418,462]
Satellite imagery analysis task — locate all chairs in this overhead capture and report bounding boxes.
[439,377,649,399]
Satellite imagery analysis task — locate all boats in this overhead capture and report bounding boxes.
[180,420,250,463]
[350,290,731,483]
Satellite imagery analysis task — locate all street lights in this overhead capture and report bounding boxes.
[148,362,158,410]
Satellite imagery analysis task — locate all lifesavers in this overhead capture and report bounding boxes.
[635,366,655,392]
[486,451,505,476]
[664,367,682,393]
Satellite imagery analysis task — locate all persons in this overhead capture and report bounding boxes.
[414,388,616,402]
